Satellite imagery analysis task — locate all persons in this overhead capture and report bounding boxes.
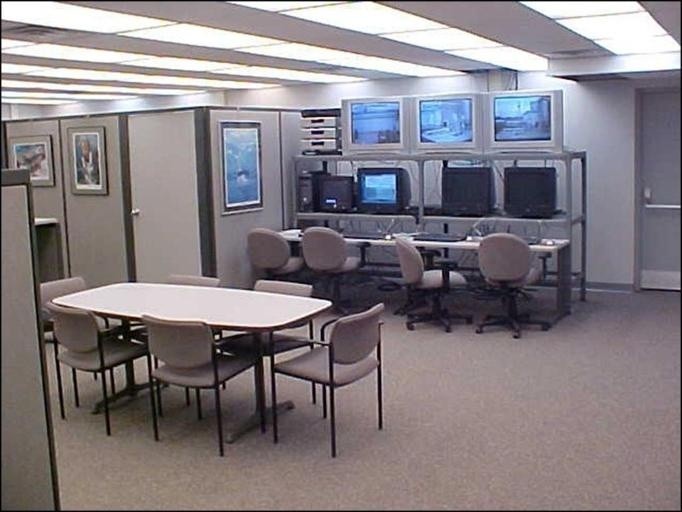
[78,139,99,185]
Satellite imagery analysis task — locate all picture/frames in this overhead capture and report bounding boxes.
[8,135,56,188]
[216,119,264,215]
[67,126,108,196]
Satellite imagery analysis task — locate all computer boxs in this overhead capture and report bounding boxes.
[297,169,328,213]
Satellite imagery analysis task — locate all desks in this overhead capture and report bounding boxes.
[51,281,329,439]
[281,223,569,308]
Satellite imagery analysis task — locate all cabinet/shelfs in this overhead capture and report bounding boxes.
[291,152,588,314]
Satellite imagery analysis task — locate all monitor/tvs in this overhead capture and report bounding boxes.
[441,167,497,217]
[318,175,355,213]
[503,167,557,218]
[347,99,405,155]
[358,168,412,214]
[489,93,556,154]
[415,96,476,155]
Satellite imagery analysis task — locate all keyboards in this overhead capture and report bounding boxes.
[343,233,383,239]
[414,233,467,242]
[522,236,539,245]
[298,227,344,237]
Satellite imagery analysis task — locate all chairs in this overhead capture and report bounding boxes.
[39,277,108,380]
[393,237,466,333]
[217,280,315,415]
[270,304,386,458]
[46,297,141,436]
[248,227,301,279]
[302,227,370,314]
[466,232,549,337]
[168,274,219,288]
[141,313,267,456]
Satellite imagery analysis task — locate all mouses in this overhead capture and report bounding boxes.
[541,239,553,246]
[384,234,392,240]
